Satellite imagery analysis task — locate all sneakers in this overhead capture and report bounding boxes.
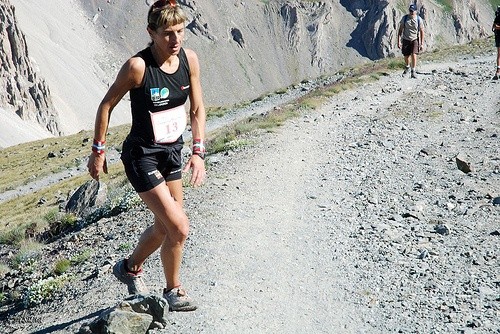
[113,259,150,295]
[163,285,198,312]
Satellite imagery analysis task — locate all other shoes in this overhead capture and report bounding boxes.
[411,70,417,78]
[492,71,500,82]
[402,66,410,78]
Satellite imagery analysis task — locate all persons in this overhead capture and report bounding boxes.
[85,0,208,311]
[397,4,425,79]
[492,6,500,80]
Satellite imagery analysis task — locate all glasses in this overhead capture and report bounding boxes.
[147,0,183,24]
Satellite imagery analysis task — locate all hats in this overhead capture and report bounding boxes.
[409,4,417,12]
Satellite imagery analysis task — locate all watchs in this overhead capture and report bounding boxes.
[193,153,205,160]
